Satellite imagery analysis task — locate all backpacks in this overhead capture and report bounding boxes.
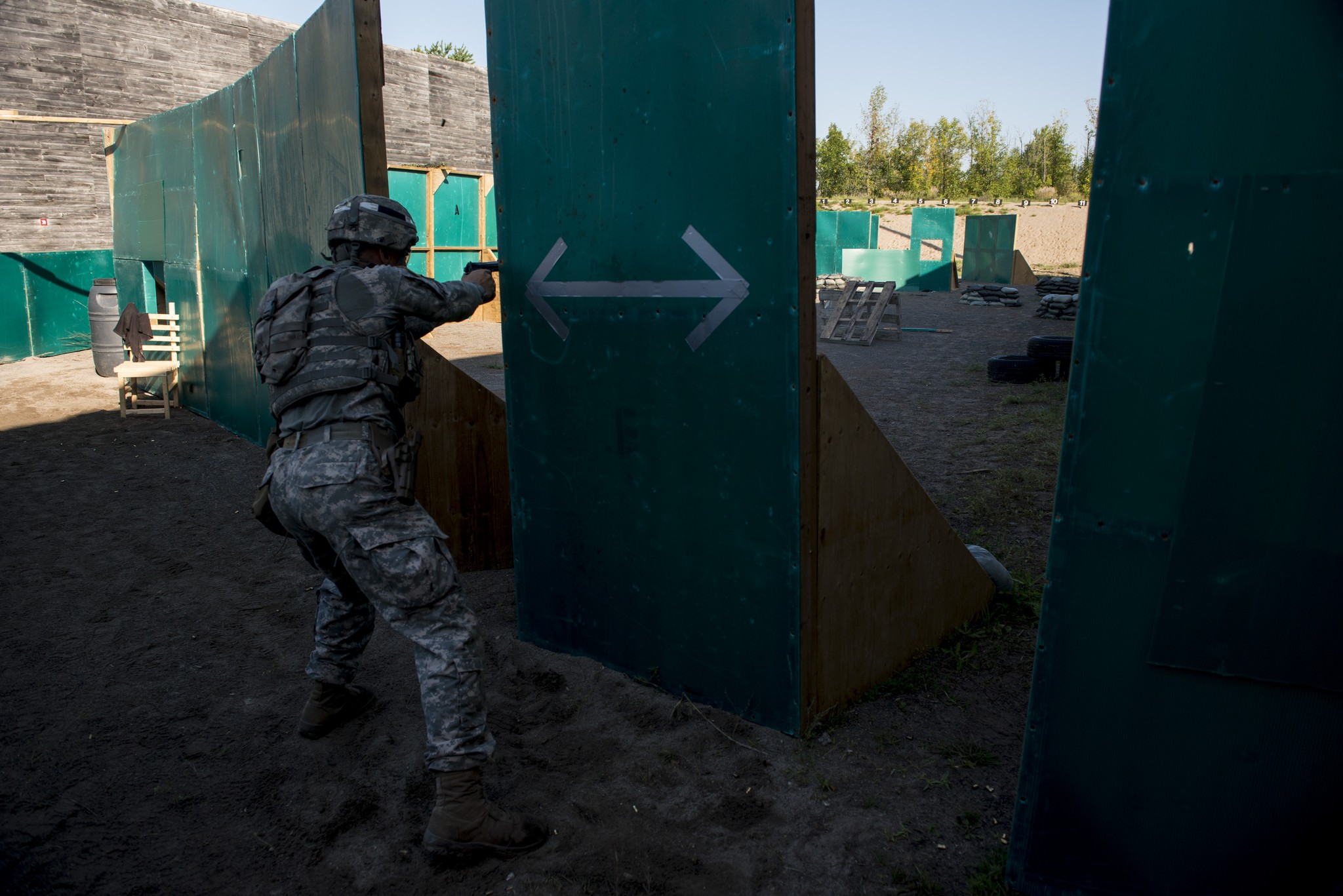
[253,265,335,387]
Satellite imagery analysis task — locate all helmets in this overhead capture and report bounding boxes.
[325,194,419,252]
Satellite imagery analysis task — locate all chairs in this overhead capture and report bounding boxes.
[113,300,181,420]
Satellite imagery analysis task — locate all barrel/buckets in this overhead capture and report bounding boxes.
[88,279,126,378]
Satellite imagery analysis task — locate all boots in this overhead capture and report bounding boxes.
[296,677,378,742]
[422,769,554,856]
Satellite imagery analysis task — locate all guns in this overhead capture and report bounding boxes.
[464,262,498,273]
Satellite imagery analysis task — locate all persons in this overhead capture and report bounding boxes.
[251,193,552,861]
